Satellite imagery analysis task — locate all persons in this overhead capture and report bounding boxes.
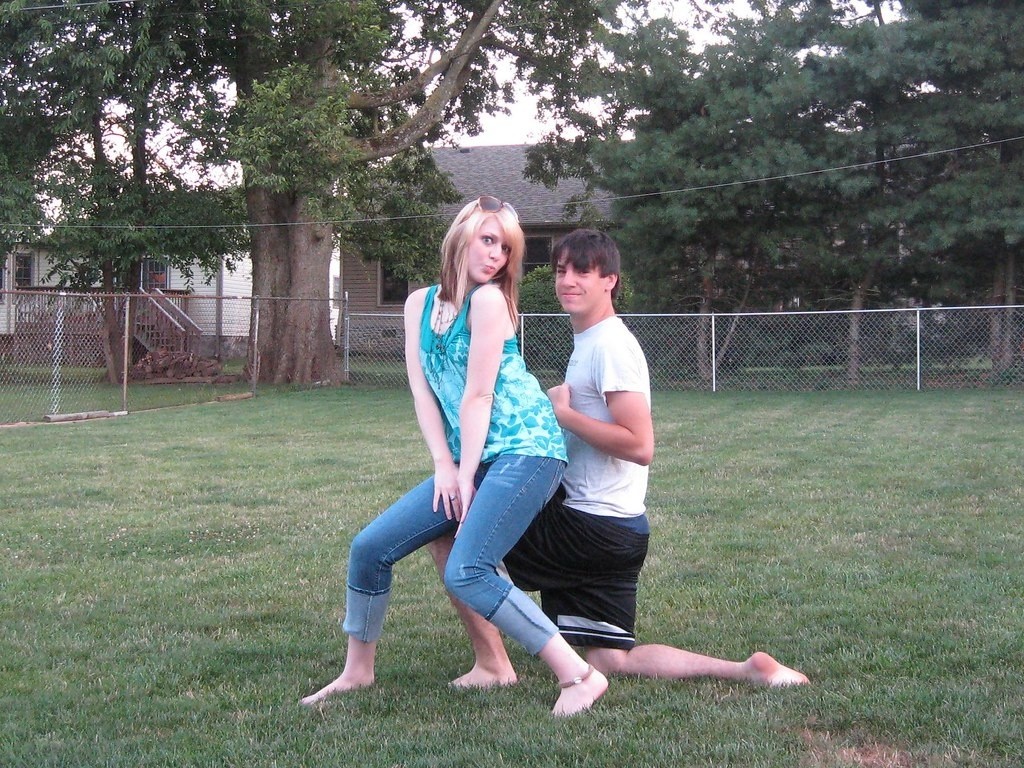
[299,196,608,716]
[427,230,811,689]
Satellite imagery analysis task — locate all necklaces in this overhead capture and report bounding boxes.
[429,300,456,389]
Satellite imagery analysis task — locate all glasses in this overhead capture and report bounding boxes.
[458,195,518,225]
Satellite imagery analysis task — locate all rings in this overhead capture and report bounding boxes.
[450,495,458,501]
